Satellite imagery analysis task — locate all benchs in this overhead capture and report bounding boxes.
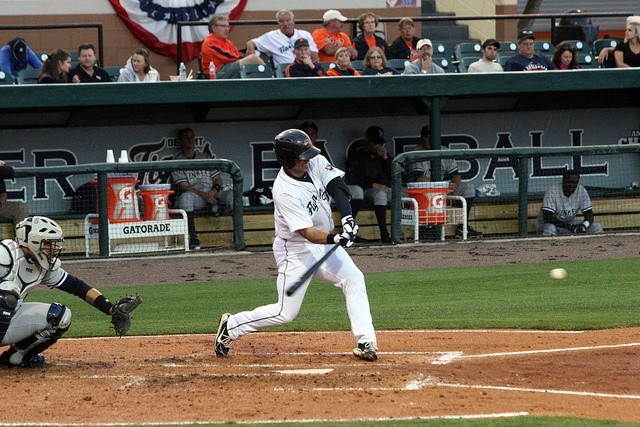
[0,186,640,256]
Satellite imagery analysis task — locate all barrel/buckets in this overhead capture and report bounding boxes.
[408,182,454,225]
[93,171,141,224]
[138,184,174,220]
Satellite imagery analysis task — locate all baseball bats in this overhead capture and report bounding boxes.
[286,242,339,296]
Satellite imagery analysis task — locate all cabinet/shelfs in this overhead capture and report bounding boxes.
[401,194,470,244]
[82,208,190,259]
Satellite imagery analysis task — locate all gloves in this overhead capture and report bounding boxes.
[334,233,353,247]
[341,215,359,247]
[569,224,587,233]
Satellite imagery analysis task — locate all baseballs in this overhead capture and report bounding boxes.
[549,267,567,280]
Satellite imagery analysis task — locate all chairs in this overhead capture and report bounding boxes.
[577,54,599,69]
[319,62,337,73]
[240,49,275,72]
[350,60,367,75]
[532,41,555,56]
[494,42,519,59]
[240,63,277,77]
[546,54,555,63]
[195,51,210,79]
[498,56,517,70]
[593,39,620,56]
[17,68,45,82]
[278,63,292,77]
[68,51,81,69]
[432,58,455,73]
[460,56,481,72]
[571,40,590,55]
[101,64,124,80]
[454,42,484,59]
[431,44,451,61]
[0,71,16,84]
[34,53,51,62]
[388,58,411,73]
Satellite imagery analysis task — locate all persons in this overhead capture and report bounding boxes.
[296,122,373,244]
[552,41,579,68]
[504,29,559,70]
[200,16,265,81]
[350,12,392,58]
[599,15,640,67]
[468,38,504,73]
[536,168,604,237]
[214,126,377,361]
[402,38,446,74]
[389,16,422,57]
[118,48,161,82]
[37,47,80,83]
[408,125,485,239]
[246,9,321,77]
[322,47,360,76]
[1,216,141,368]
[169,127,245,251]
[312,8,358,61]
[347,126,406,242]
[69,43,112,83]
[360,46,400,75]
[0,37,43,85]
[289,38,329,76]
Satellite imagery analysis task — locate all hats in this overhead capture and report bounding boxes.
[294,39,309,48]
[366,126,386,143]
[517,29,535,45]
[323,9,348,22]
[416,38,433,50]
[483,39,500,49]
[13,41,26,61]
[422,126,440,136]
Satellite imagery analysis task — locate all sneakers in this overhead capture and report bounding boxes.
[456,223,483,239]
[189,237,200,250]
[214,313,234,357]
[353,343,377,361]
[0,350,45,368]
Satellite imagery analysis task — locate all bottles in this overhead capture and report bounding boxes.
[209,61,216,80]
[179,62,186,81]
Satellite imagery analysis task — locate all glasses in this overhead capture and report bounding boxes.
[369,56,382,58]
[214,24,230,26]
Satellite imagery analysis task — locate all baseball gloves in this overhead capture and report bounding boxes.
[110,292,143,339]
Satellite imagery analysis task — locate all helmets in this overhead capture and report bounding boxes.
[16,216,63,271]
[274,129,320,169]
